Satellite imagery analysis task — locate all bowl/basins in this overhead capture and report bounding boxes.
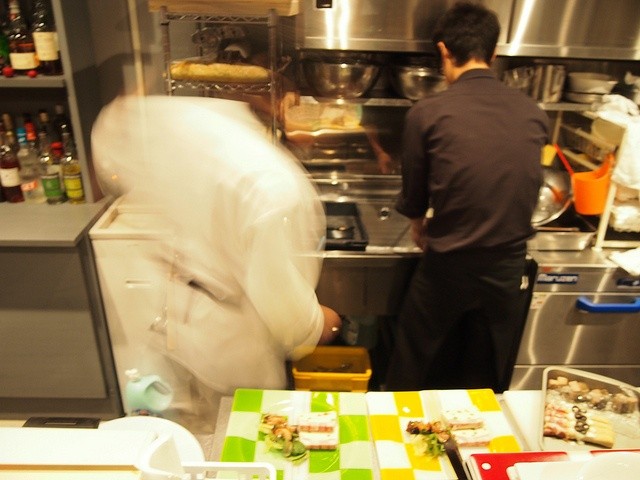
[501,63,567,103]
[399,66,447,99]
[303,59,380,97]
[567,71,618,94]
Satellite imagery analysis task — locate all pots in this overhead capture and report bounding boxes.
[530,171,575,227]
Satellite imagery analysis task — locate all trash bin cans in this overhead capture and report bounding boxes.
[291,346,373,392]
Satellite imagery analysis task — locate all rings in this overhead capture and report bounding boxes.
[332,326,339,332]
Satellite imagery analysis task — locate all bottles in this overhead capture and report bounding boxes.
[32,0,64,76]
[16,127,46,204]
[3,0,41,75]
[39,113,57,143]
[36,132,65,205]
[53,105,66,129]
[25,122,39,156]
[1,124,25,203]
[61,125,86,205]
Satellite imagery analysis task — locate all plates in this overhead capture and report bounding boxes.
[98,415,206,479]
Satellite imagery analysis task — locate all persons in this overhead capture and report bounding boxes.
[89,95,343,389]
[380,3,552,389]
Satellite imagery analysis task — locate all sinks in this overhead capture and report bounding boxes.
[359,200,418,246]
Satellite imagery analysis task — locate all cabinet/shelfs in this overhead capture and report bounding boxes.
[147,0,303,146]
[309,92,595,205]
[0,0,151,425]
